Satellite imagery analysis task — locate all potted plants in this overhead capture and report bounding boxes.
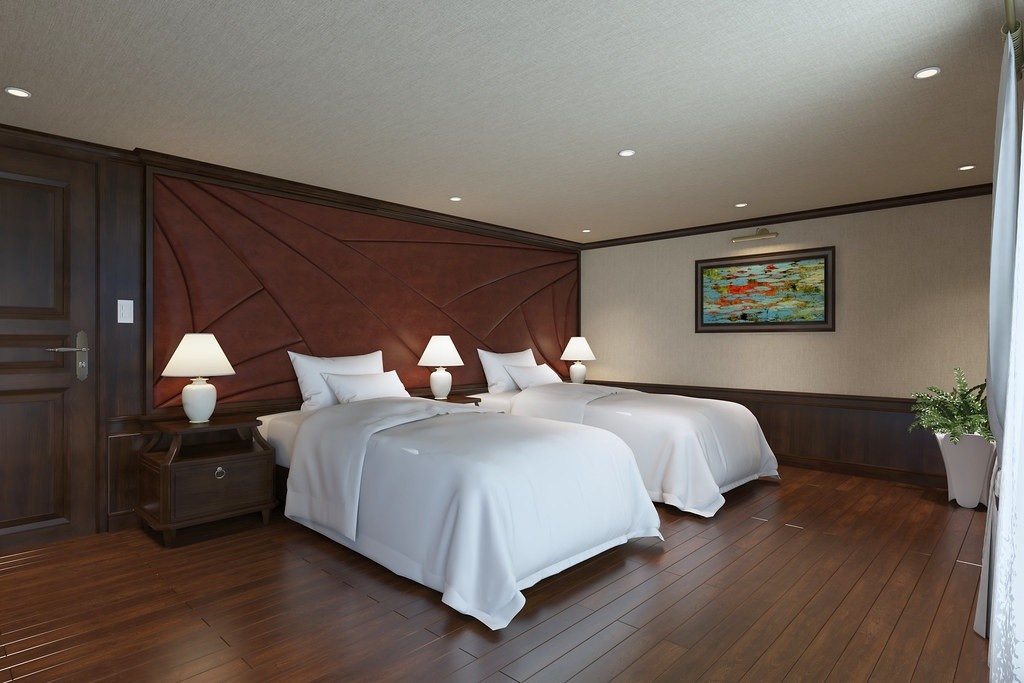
[908,367,997,508]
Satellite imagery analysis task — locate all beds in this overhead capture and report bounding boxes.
[464,382,781,519]
[256,397,664,633]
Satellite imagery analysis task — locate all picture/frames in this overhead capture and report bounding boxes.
[695,246,836,334]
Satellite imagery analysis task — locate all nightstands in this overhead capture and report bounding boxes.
[135,417,275,546]
[428,395,481,406]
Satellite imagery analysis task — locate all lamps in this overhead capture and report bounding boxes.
[560,337,597,384]
[161,332,236,424]
[417,336,465,399]
[732,227,779,243]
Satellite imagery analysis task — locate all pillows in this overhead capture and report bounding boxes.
[503,362,562,391]
[319,369,410,405]
[287,350,384,411]
[477,348,537,394]
